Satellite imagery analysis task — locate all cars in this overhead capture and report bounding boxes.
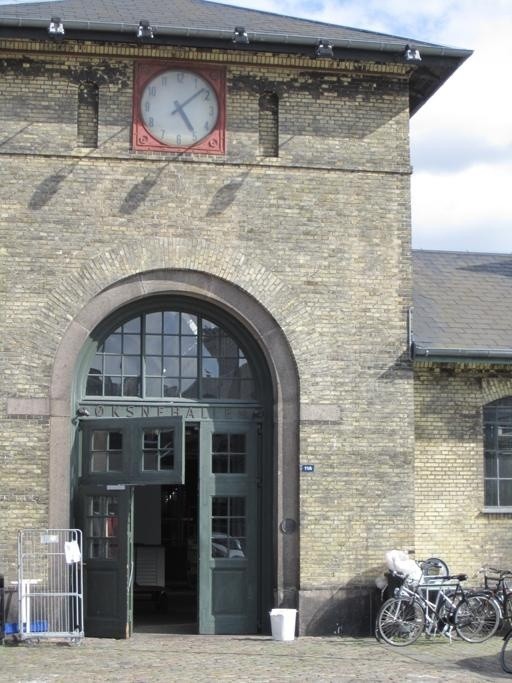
[211,531,244,557]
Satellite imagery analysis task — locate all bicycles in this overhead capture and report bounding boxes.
[373,557,512,676]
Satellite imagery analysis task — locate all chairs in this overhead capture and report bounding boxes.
[87,540,167,601]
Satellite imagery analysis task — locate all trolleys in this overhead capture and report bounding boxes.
[15,527,86,646]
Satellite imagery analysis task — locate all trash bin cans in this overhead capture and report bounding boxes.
[269,608,299,641]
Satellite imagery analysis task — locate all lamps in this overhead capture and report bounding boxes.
[133,18,159,42]
[314,35,338,61]
[231,24,251,43]
[401,41,425,67]
[49,14,68,35]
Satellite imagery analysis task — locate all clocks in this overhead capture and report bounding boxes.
[127,57,227,157]
